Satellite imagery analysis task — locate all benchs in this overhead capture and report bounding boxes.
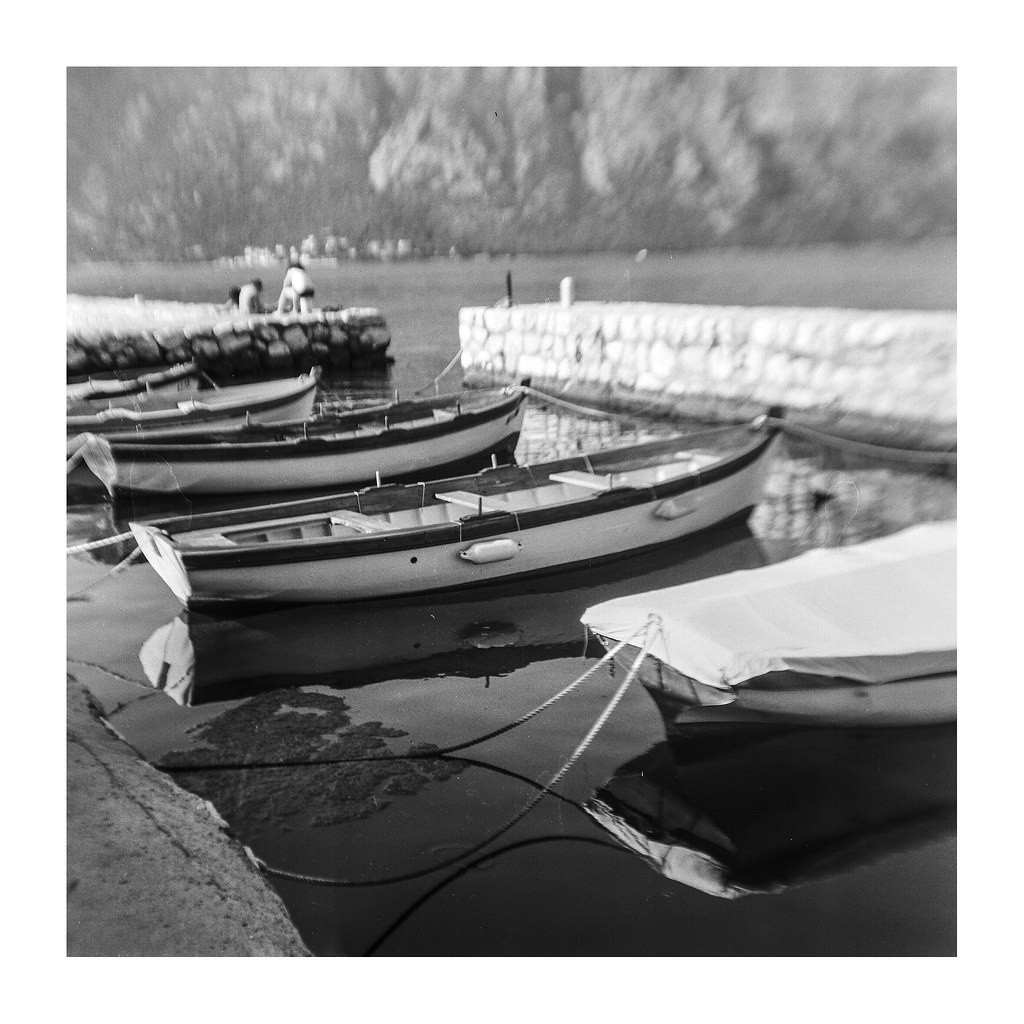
[435,490,519,513]
[549,470,618,490]
[176,400,206,414]
[358,421,387,434]
[433,408,458,418]
[329,509,396,533]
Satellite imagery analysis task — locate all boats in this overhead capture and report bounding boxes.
[124,405,782,611]
[586,718,958,899]
[137,525,776,707]
[67,362,318,439]
[66,358,198,412]
[580,517,958,732]
[67,378,532,506]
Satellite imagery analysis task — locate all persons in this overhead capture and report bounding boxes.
[223,261,314,316]
